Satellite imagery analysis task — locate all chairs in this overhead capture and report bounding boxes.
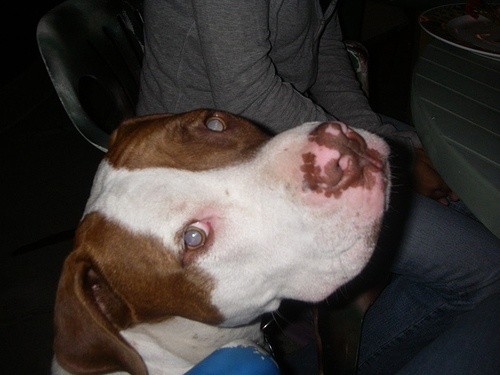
[35,0,399,375]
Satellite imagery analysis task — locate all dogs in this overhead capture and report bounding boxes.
[50,106,391,375]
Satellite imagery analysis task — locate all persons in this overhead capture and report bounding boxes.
[136,0,500,375]
